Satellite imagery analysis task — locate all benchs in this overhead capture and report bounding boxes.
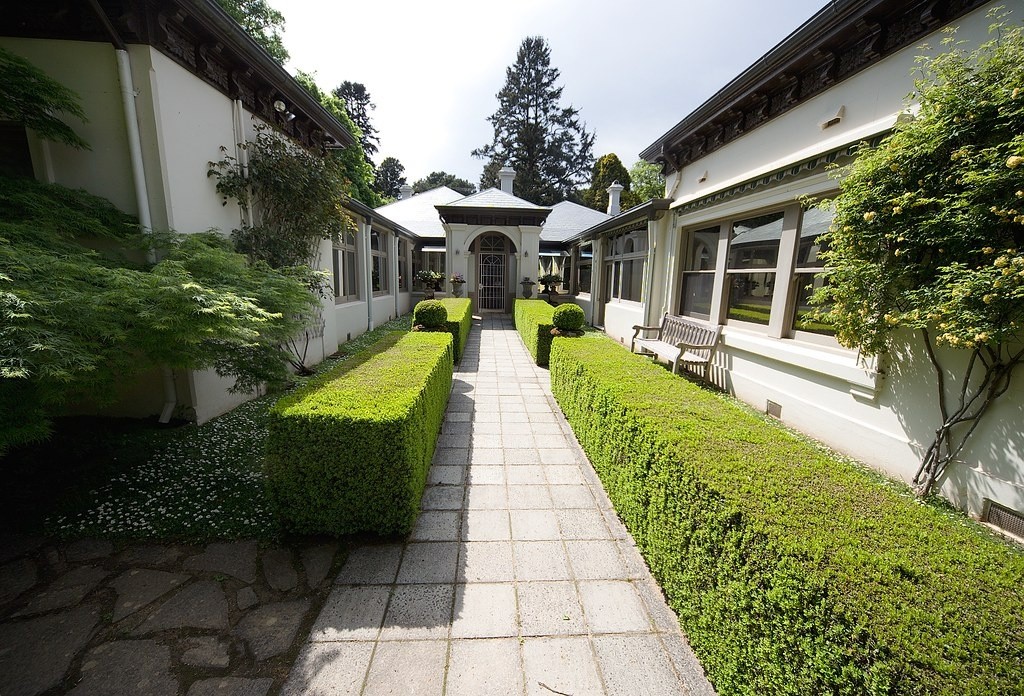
[630,312,723,386]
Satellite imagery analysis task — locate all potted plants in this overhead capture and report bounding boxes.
[448,272,466,298]
[548,274,562,295]
[415,270,441,301]
[548,303,586,369]
[540,274,552,294]
[412,299,448,333]
